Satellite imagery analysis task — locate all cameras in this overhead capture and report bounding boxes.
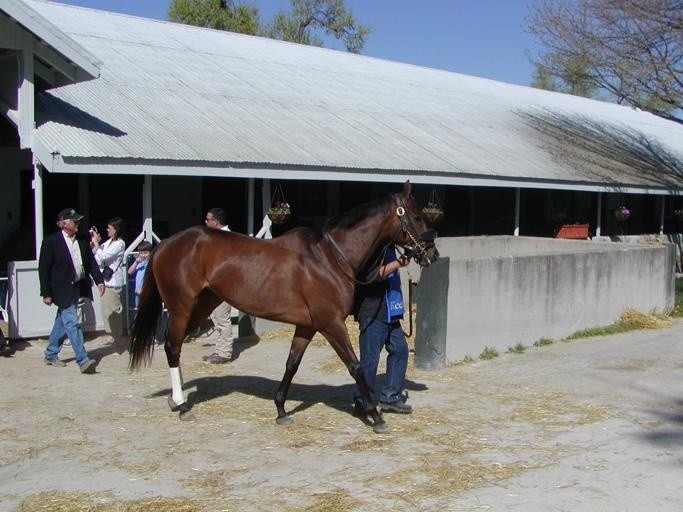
[91,225,98,234]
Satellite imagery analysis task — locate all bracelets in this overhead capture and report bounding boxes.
[98,284,105,286]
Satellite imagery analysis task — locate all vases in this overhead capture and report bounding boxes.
[424,214,440,222]
[616,214,629,220]
[267,213,287,223]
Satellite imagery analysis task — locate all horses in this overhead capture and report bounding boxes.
[127,179,440,434]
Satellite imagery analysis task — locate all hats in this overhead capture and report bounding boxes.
[57,208,84,222]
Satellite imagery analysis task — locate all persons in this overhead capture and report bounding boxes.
[38,209,105,373]
[353,242,412,417]
[202,208,234,363]
[90,216,126,355]
[128,241,158,348]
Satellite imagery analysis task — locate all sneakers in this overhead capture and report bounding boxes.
[203,353,232,364]
[44,356,65,367]
[355,405,382,426]
[79,359,96,373]
[378,399,412,414]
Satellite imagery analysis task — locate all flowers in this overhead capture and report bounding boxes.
[268,201,291,214]
[422,201,441,213]
[616,205,628,213]
